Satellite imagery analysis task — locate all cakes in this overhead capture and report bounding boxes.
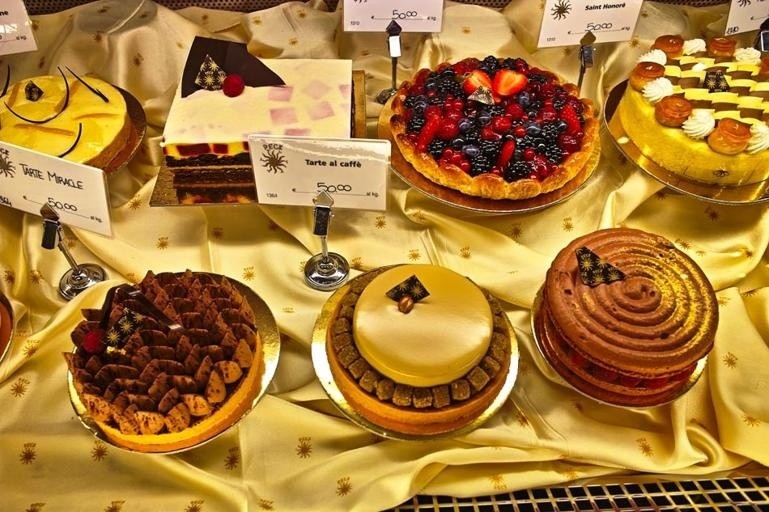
[534,228,719,407]
[159,35,355,190]
[620,36,769,187]
[61,270,262,452]
[0,63,132,173]
[0,299,13,357]
[325,264,512,436]
[390,55,600,201]
